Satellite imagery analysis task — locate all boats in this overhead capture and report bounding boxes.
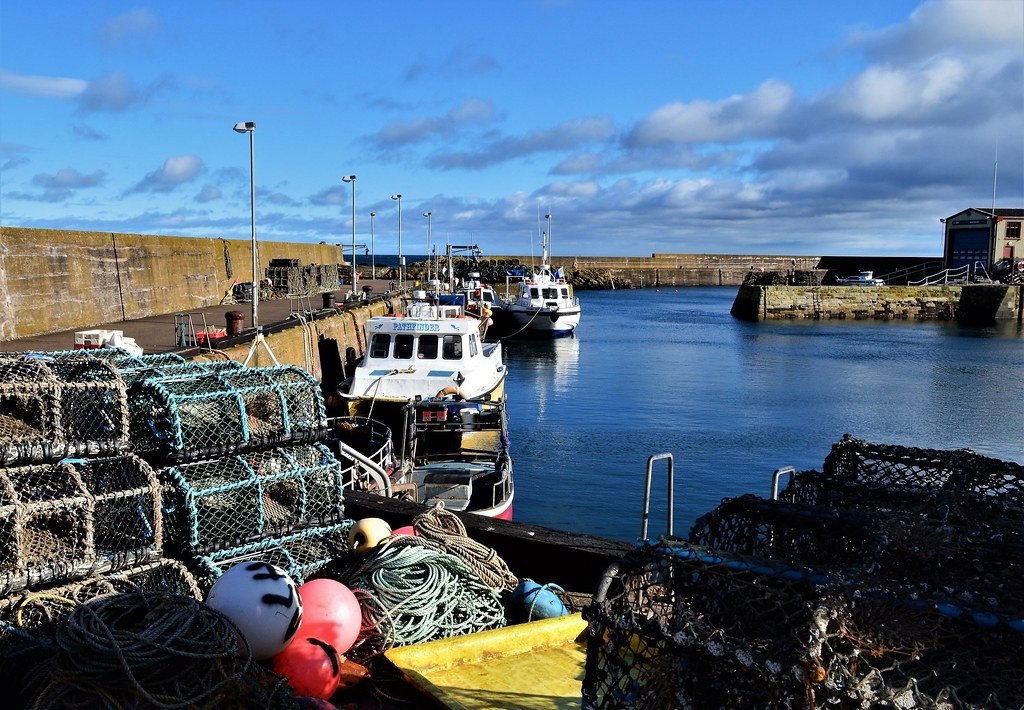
[336,291,508,441]
[372,394,514,522]
[297,415,407,498]
[835,271,885,286]
[499,232,581,339]
[406,272,508,354]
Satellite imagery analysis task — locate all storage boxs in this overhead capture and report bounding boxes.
[195,331,226,344]
[74,330,145,358]
[423,408,448,422]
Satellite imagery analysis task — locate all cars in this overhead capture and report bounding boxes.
[989,257,1024,284]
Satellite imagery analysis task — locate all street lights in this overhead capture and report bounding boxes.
[371,213,375,280]
[423,213,430,282]
[391,195,402,288]
[232,122,258,327]
[342,175,356,295]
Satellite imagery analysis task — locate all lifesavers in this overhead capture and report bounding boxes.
[384,313,405,317]
[435,387,468,403]
[556,282,567,285]
[526,281,538,285]
[449,314,474,319]
